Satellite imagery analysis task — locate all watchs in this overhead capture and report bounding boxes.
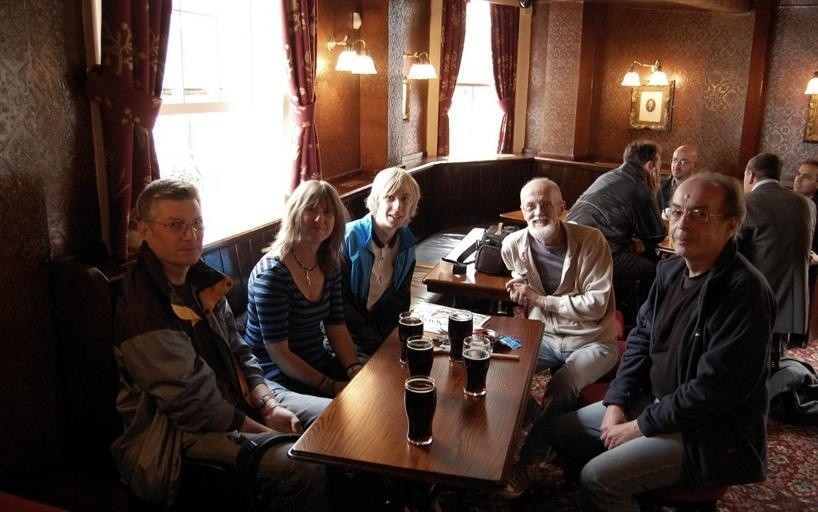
[255,394,273,409]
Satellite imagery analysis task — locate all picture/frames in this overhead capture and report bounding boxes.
[629,80,675,133]
[803,94,818,144]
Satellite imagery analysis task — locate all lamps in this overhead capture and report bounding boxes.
[328,35,378,75]
[403,52,439,80]
[620,59,641,86]
[646,58,670,86]
[803,71,818,95]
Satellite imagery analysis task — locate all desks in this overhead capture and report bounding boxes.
[422,227,519,317]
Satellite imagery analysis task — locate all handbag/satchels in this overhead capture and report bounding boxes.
[457,222,517,276]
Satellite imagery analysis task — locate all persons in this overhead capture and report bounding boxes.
[562,140,667,281]
[338,166,420,342]
[792,160,818,267]
[243,179,363,430]
[110,179,333,512]
[734,152,818,335]
[657,143,699,213]
[496,176,619,500]
[552,171,778,511]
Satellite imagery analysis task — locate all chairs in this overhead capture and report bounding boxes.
[632,331,790,511]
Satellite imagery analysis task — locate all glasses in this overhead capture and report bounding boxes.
[670,158,690,166]
[660,206,724,224]
[520,201,560,211]
[144,218,205,234]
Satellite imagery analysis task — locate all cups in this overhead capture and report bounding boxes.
[406,335,434,377]
[404,375,438,447]
[462,336,493,397]
[398,312,423,364]
[448,310,473,363]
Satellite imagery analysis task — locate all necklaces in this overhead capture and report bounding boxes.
[290,247,318,287]
